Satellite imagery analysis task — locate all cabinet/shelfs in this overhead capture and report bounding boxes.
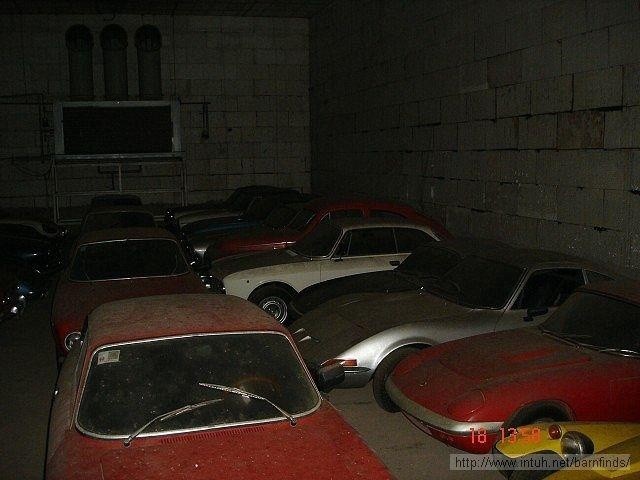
[51,159,188,227]
